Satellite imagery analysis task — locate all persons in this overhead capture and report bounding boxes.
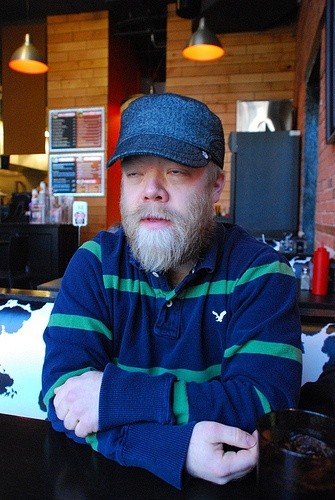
[41,94,303,489]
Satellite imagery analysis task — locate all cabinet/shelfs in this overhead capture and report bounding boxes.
[0,223,78,288]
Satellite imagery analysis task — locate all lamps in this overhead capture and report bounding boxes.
[181,19,224,61]
[8,0,49,75]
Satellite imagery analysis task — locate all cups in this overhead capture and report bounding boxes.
[253,408,335,500]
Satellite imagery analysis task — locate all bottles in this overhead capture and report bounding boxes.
[312,241,331,296]
[300,267,312,290]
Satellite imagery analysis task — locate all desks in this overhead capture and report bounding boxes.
[0,413,335,500]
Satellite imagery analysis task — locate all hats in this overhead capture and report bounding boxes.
[105,92,225,169]
